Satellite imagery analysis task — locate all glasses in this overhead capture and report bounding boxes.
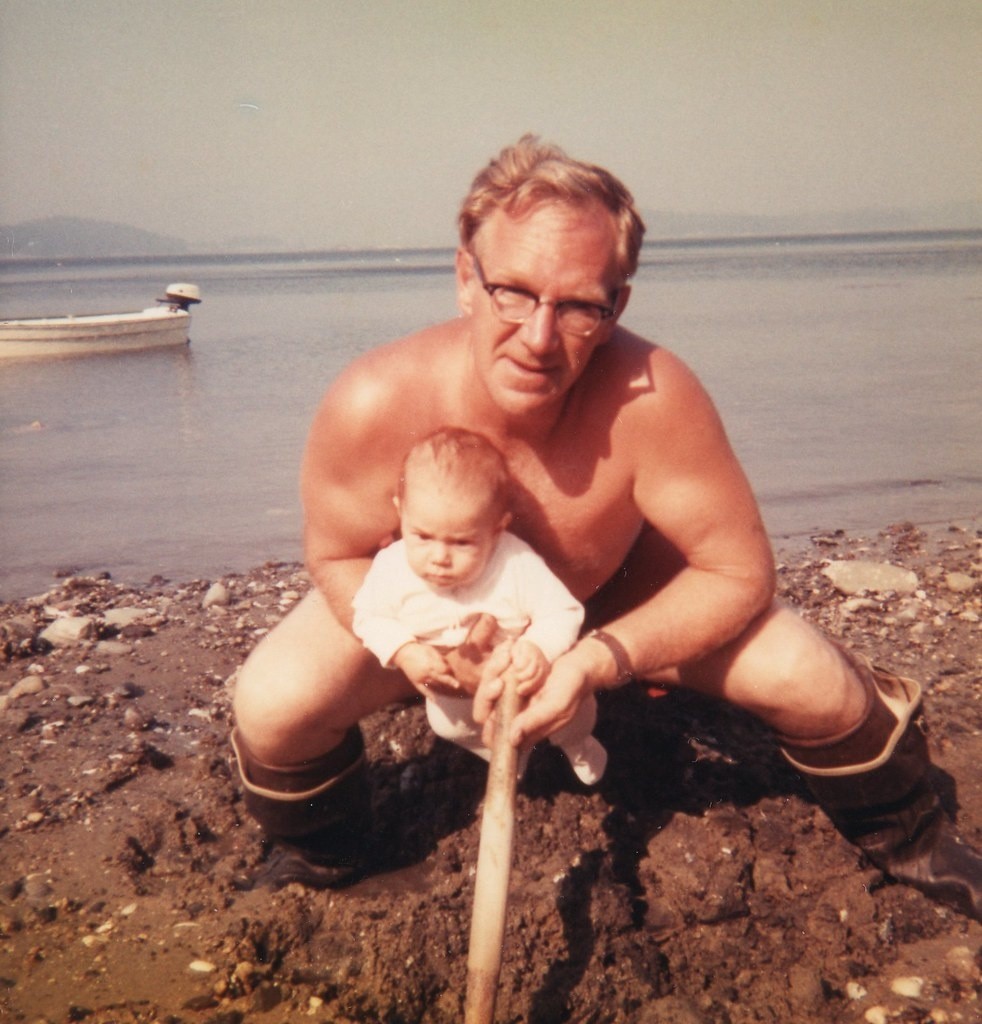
[470,254,622,339]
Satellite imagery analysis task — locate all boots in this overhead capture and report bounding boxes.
[229,724,376,892]
[760,635,982,924]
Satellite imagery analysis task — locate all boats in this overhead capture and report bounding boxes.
[0,281,202,368]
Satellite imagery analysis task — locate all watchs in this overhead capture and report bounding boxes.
[590,627,632,691]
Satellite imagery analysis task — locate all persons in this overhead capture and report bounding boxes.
[228,133,982,928]
[352,427,607,785]
